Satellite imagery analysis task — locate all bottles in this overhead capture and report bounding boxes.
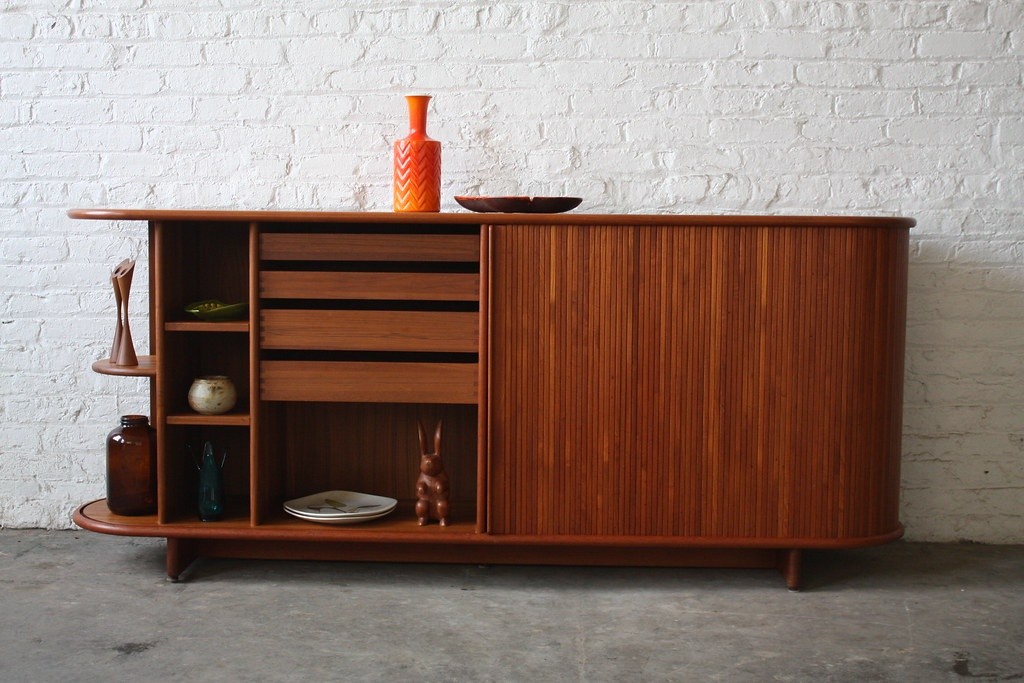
[106,415,156,516]
[393,95,442,212]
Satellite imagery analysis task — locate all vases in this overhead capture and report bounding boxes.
[393,94,442,213]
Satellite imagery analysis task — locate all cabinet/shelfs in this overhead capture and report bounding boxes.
[68,211,918,592]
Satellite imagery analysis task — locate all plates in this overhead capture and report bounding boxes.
[454,196,582,213]
[283,490,398,523]
[184,297,249,321]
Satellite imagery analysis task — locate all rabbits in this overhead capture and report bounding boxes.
[415,419,452,527]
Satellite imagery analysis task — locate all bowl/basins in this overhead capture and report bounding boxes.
[188,375,236,414]
